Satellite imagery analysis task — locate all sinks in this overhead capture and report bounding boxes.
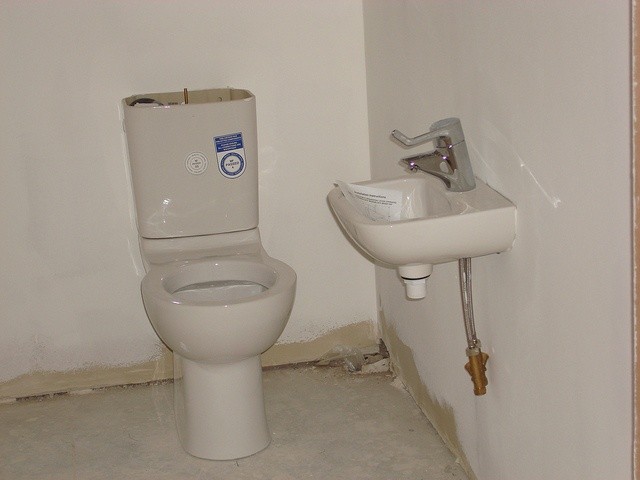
[327,173,518,279]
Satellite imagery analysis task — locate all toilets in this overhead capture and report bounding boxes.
[121,87,297,462]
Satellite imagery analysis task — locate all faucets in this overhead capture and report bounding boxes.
[391,117,476,193]
